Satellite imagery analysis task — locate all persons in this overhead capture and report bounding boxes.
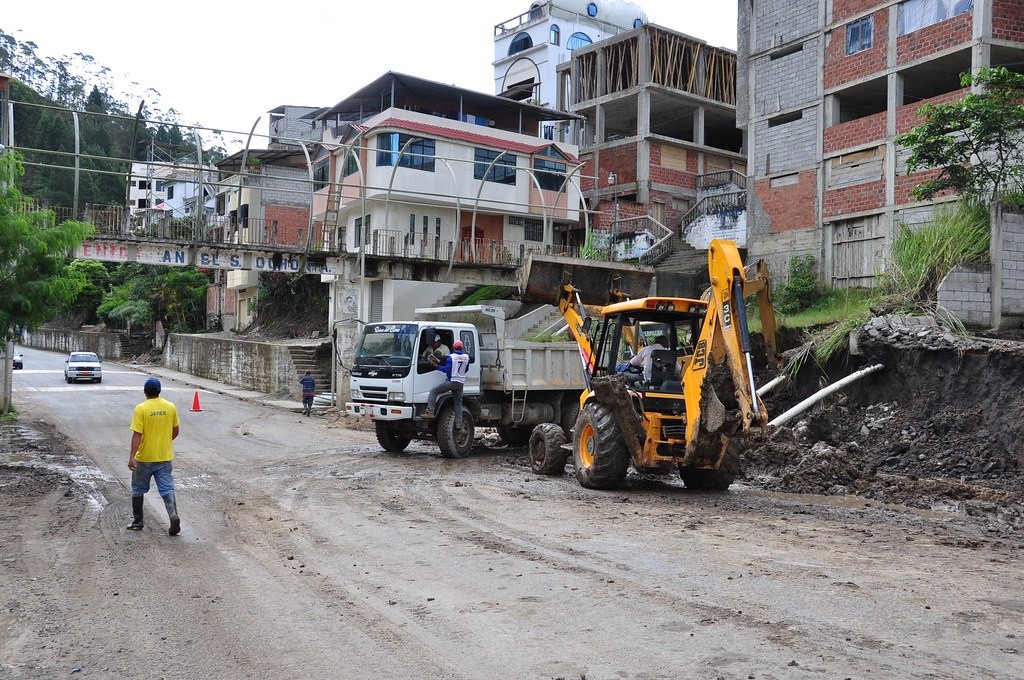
[299,369,316,418]
[421,334,451,364]
[125,377,182,537]
[420,339,470,430]
[629,334,683,386]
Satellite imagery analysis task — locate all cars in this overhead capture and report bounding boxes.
[64,352,103,383]
[12,351,24,369]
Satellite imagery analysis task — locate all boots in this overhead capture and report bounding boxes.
[301,403,307,415]
[163,494,180,536]
[126,496,144,531]
[305,403,311,416]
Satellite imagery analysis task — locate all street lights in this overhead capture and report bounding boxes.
[608,171,618,255]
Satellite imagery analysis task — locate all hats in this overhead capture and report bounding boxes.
[452,340,463,348]
[144,377,161,390]
[432,334,440,342]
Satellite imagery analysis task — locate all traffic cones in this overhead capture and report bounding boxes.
[190,391,203,413]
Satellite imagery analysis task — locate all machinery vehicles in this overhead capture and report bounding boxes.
[528,239,885,494]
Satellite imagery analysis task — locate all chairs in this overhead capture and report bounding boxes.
[650,350,677,385]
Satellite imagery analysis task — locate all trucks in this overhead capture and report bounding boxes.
[331,305,622,460]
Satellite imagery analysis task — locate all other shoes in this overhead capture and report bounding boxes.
[420,412,434,419]
[455,421,462,428]
